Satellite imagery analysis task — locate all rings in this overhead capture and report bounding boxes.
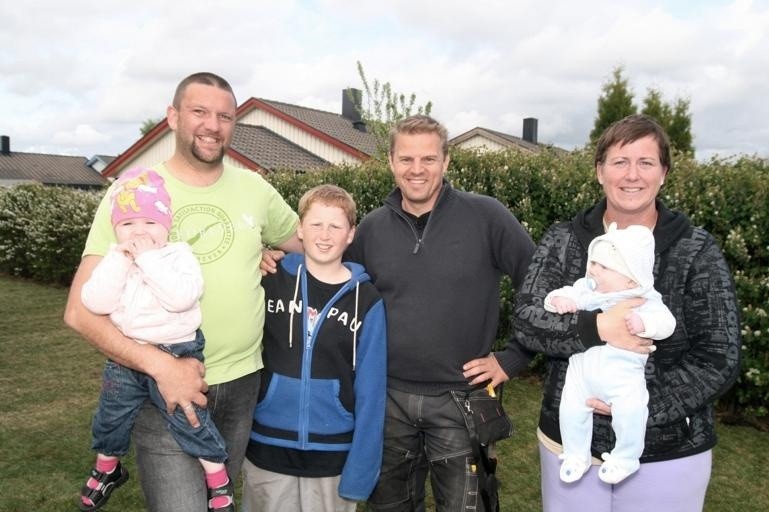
[183,403,192,412]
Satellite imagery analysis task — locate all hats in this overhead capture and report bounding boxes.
[591,240,637,284]
[111,166,172,232]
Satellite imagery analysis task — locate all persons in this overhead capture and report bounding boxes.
[239,181,390,512]
[60,71,304,512]
[80,166,237,512]
[260,115,539,512]
[543,221,678,487]
[508,113,744,512]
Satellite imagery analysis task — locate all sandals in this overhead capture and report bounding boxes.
[206,474,235,512]
[78,460,130,511]
